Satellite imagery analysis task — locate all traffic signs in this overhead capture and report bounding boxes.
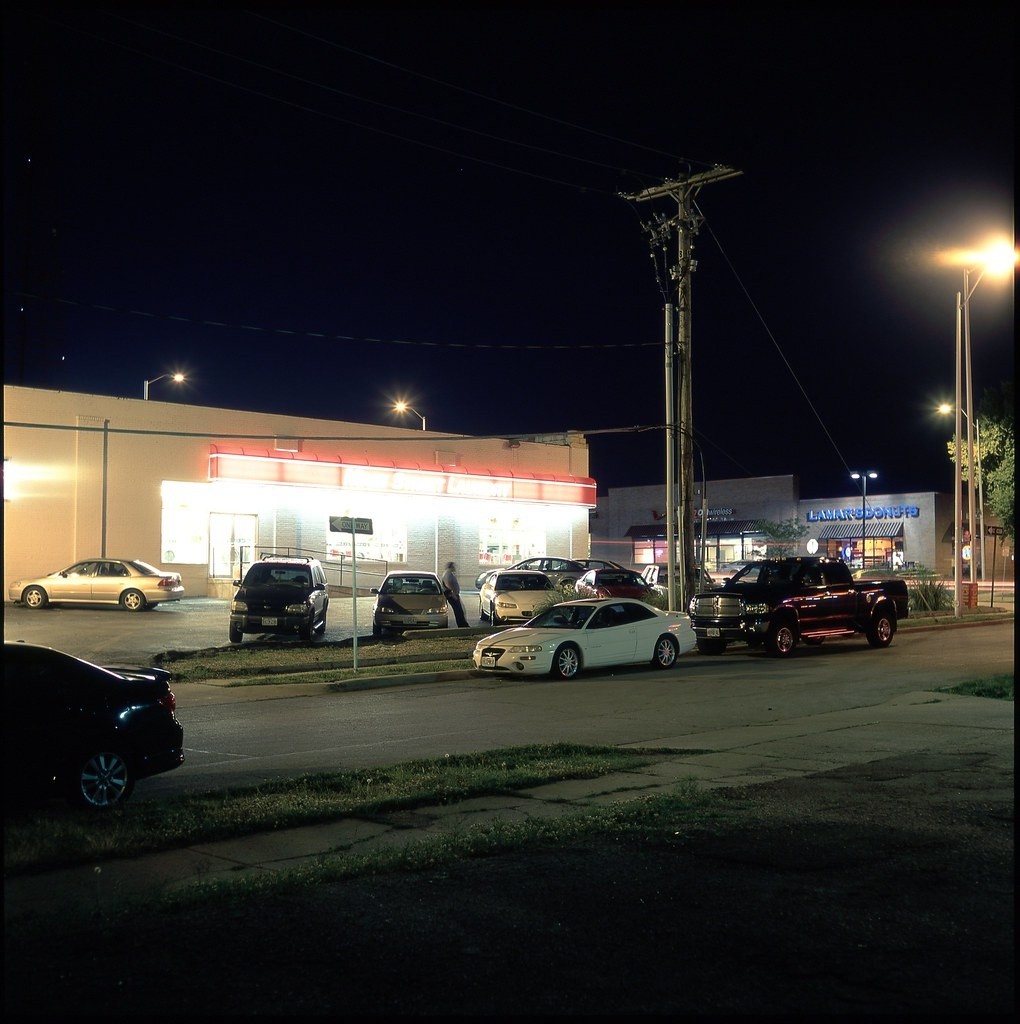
[330,516,373,534]
[988,526,1004,536]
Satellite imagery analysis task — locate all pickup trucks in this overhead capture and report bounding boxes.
[690,557,909,659]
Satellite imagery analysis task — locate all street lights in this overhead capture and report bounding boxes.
[397,405,425,431]
[144,374,182,400]
[850,470,879,569]
[940,407,986,582]
[955,253,1011,619]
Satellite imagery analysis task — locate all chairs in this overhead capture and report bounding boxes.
[295,576,305,583]
[422,580,433,589]
[260,574,273,584]
[101,567,117,576]
[499,579,538,591]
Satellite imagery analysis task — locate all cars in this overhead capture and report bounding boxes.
[554,559,624,570]
[4,641,185,816]
[475,556,591,590]
[637,564,722,599]
[477,570,563,627]
[472,596,698,681]
[575,569,655,600]
[9,558,185,612]
[369,571,453,636]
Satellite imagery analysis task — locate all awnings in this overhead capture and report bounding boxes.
[623,518,765,542]
[817,522,904,541]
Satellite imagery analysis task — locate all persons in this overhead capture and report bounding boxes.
[599,611,612,626]
[768,573,776,584]
[571,609,578,622]
[441,561,471,628]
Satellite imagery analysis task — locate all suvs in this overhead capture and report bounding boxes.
[230,553,329,644]
